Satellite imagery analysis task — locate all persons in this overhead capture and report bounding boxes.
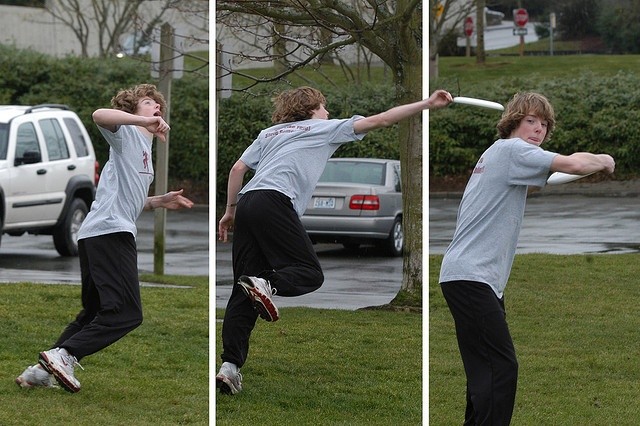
[14,85,196,392]
[215,87,453,396]
[438,91,617,426]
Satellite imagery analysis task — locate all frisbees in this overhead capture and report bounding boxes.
[546,172,595,185]
[452,96,504,112]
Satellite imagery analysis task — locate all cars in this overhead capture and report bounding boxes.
[299,157,403,256]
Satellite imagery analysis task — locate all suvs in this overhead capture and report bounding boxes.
[0,102,101,260]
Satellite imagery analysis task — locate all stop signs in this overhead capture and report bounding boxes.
[463,16,473,37]
[512,7,529,27]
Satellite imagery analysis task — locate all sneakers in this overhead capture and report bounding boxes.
[38,347,84,393]
[16,365,60,390]
[237,275,279,322]
[216,362,242,395]
